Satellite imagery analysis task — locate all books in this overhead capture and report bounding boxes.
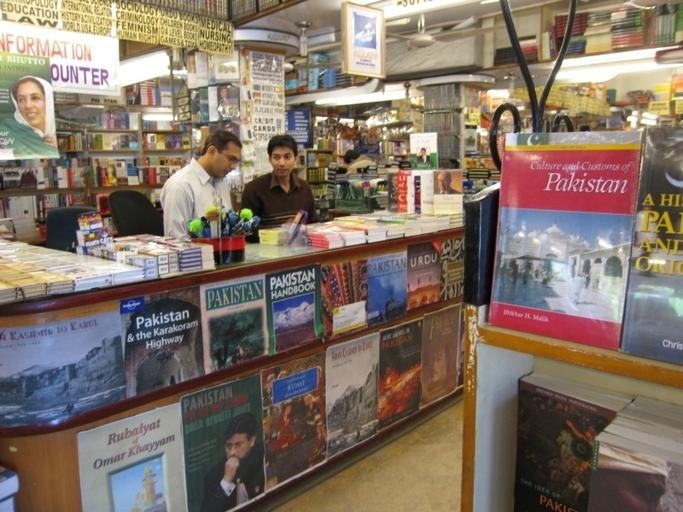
[619,125,683,367]
[512,370,682,512]
[184,45,241,124]
[486,127,645,351]
[304,132,501,252]
[537,0,683,62]
[0,236,144,305]
[0,52,60,160]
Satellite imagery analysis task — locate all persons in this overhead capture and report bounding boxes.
[243,135,319,228]
[504,255,603,293]
[259,368,332,483]
[421,341,453,403]
[10,76,60,159]
[198,409,268,512]
[160,129,244,242]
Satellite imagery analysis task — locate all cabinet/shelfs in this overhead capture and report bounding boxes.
[0,129,186,246]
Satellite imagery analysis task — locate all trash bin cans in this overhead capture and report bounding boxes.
[0,467,20,511]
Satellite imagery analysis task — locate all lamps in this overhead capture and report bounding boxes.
[294,20,312,56]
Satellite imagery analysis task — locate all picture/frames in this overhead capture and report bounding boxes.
[341,1,387,80]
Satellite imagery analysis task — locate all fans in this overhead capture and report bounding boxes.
[386,13,507,47]
